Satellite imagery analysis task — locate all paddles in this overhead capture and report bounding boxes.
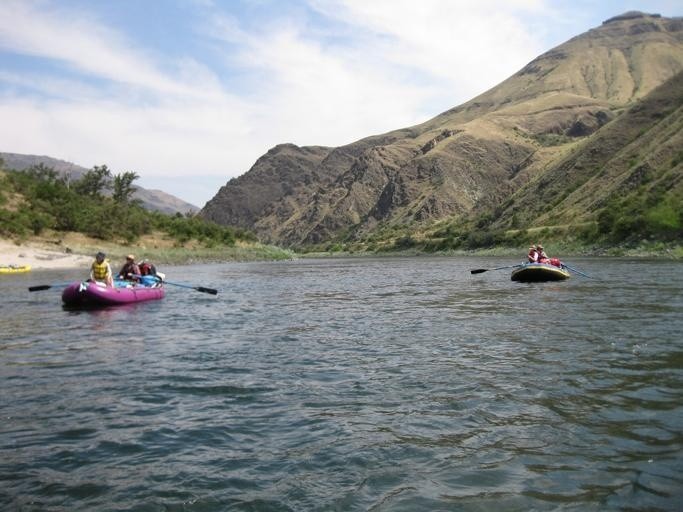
[471,265,521,274]
[28,283,71,291]
[129,275,217,295]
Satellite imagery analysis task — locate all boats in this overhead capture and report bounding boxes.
[0,263,31,274]
[62,278,165,304]
[510,260,570,282]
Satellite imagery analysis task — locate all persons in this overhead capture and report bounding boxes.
[536,244,547,264]
[527,244,540,264]
[136,258,156,286]
[88,251,112,287]
[114,256,141,283]
